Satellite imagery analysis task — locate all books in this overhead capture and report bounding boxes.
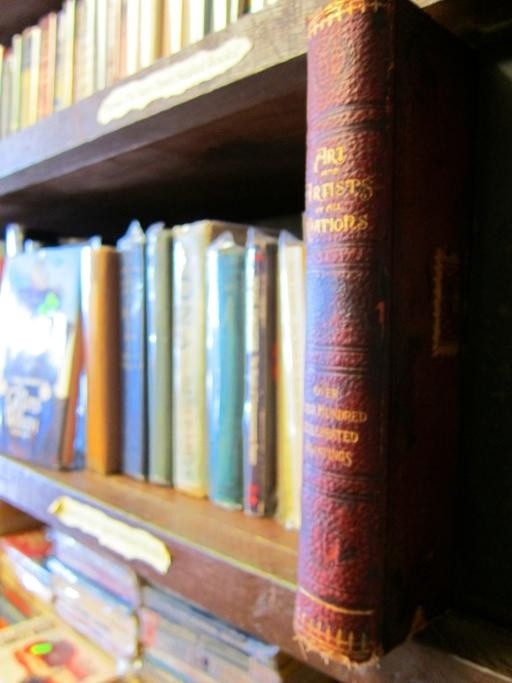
[0,0,282,136]
[1,220,311,532]
[0,533,341,682]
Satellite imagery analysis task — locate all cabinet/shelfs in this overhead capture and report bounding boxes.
[1,1,511,683]
